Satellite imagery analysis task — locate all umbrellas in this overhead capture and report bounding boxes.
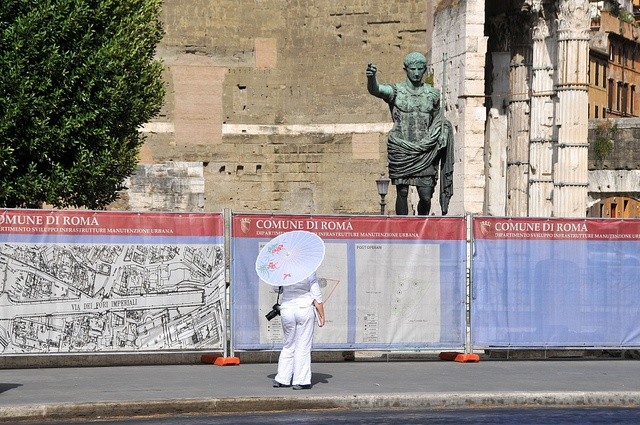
[255,230,325,286]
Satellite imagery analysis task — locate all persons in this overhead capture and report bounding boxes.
[272,271,326,390]
[366,51,454,215]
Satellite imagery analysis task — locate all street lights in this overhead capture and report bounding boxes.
[376,171,390,215]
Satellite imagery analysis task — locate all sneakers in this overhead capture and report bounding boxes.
[272,380,282,388]
[292,385,305,390]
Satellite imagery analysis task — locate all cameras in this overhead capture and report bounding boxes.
[265,304,281,321]
